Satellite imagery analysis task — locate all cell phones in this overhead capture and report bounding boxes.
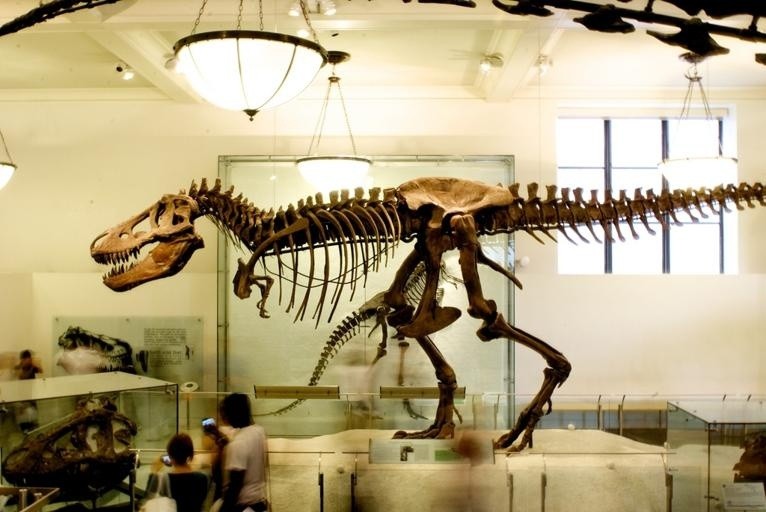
[202,418,215,426]
[159,456,171,467]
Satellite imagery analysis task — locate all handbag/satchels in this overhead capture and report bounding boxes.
[138,495,178,511]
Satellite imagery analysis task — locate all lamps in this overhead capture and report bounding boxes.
[0,130,17,190]
[656,51,739,193]
[170,0,330,121]
[297,50,373,204]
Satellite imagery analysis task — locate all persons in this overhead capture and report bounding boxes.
[206,391,272,511]
[10,349,43,433]
[143,432,212,512]
